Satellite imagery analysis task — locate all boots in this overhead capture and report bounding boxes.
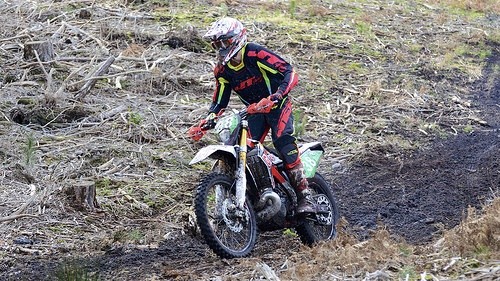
[286,163,318,215]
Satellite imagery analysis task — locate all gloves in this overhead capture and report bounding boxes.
[268,91,283,107]
[200,113,217,131]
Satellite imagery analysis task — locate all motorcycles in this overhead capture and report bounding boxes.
[189,97,340,260]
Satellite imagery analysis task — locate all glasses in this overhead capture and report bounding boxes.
[211,27,246,50]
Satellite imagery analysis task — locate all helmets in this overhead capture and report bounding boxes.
[203,17,247,66]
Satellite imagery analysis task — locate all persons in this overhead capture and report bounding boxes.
[189,16,314,212]
